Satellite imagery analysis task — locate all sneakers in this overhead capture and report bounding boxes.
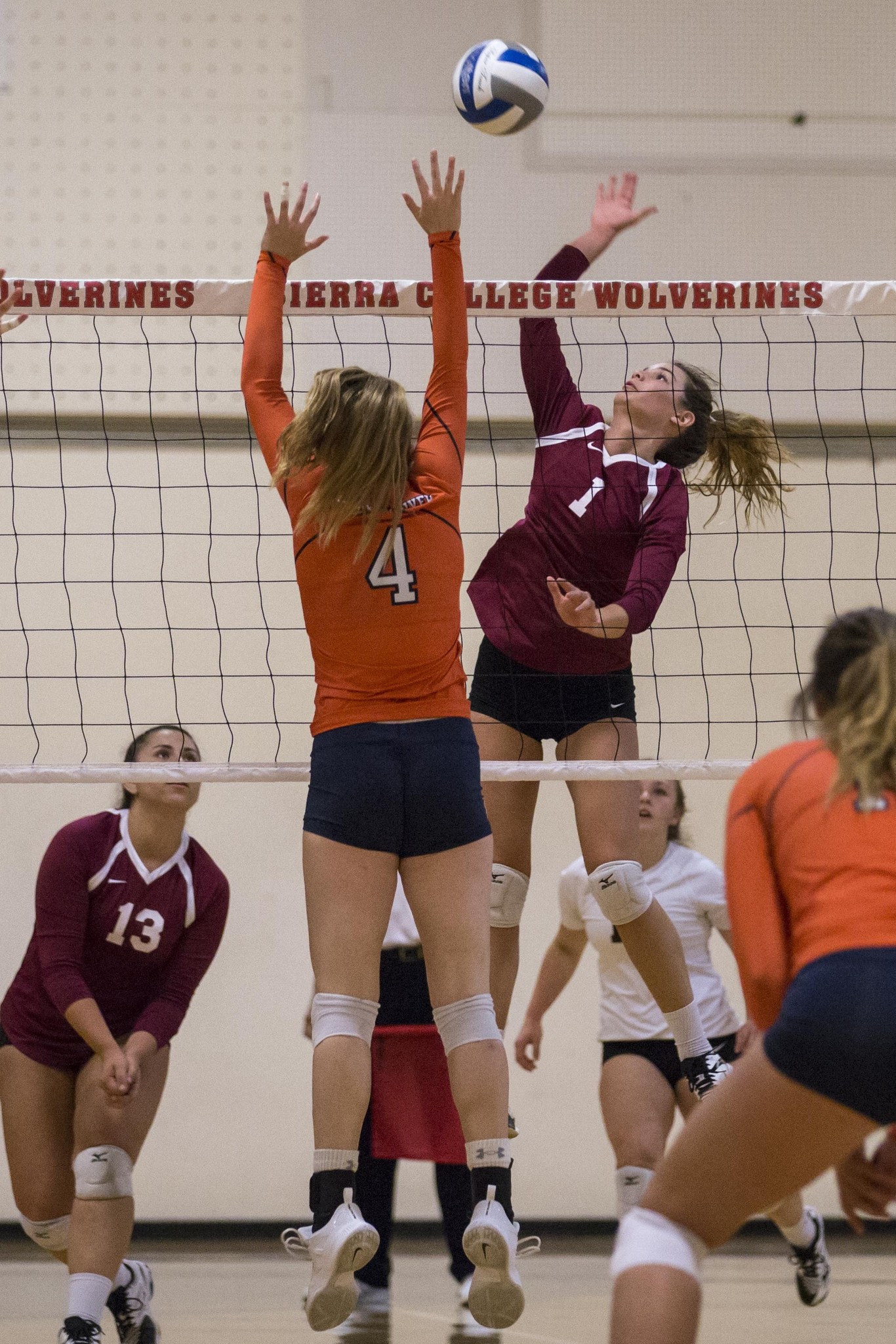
[336,1278,393,1344]
[787,1208,832,1307]
[507,1112,518,1139]
[462,1185,541,1328]
[56,1314,106,1344]
[681,1051,733,1103]
[105,1257,160,1344]
[280,1187,380,1332]
[446,1276,502,1344]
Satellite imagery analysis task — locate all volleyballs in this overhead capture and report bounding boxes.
[452,37,549,136]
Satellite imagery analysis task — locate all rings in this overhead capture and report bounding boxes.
[857,1182,867,1193]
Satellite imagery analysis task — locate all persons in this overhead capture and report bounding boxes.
[239,151,528,1330]
[0,725,230,1344]
[304,870,510,1344]
[513,756,835,1307]
[467,172,800,1101]
[609,608,896,1344]
